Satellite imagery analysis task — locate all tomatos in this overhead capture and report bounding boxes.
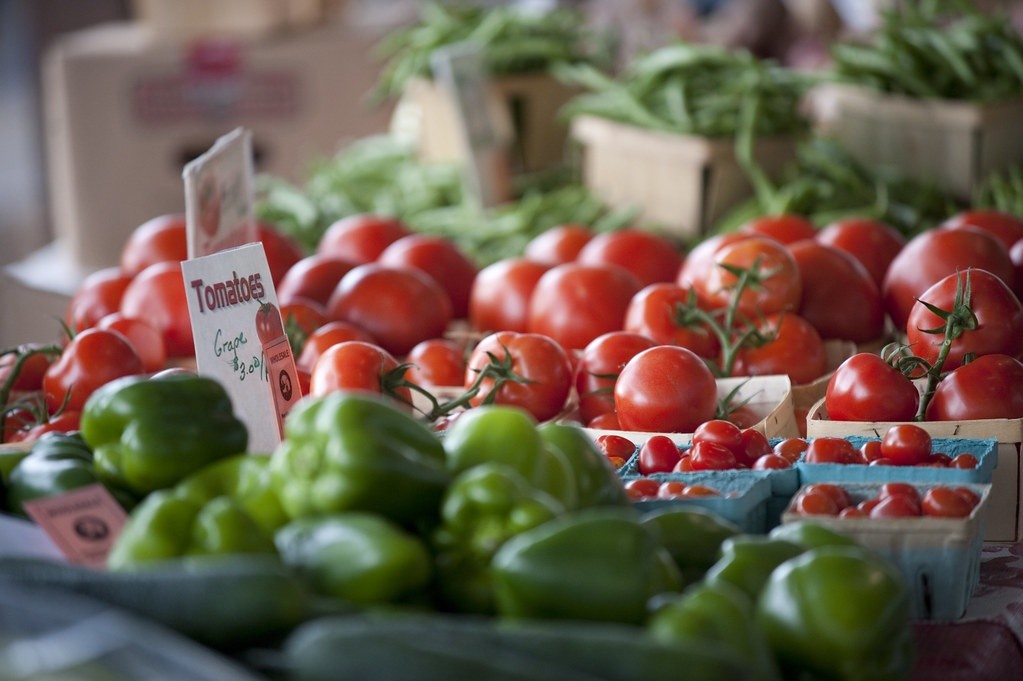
[0,202,1023,521]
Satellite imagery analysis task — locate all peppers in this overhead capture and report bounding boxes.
[9,367,913,681]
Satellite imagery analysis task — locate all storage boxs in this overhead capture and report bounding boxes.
[46,2,1022,594]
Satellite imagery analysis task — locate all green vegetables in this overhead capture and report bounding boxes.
[239,1,1023,239]
